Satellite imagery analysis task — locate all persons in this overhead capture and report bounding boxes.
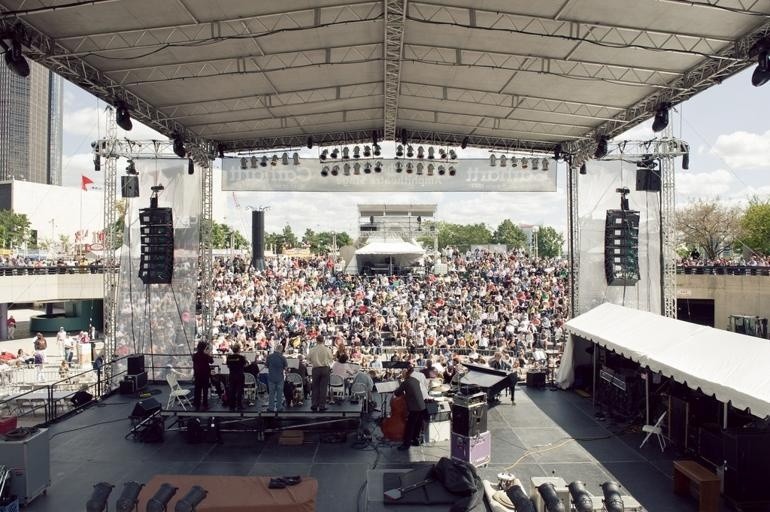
[394,367,427,450]
[266,342,290,411]
[307,335,332,412]
[674,249,770,274]
[191,340,215,409]
[119,248,569,404]
[1,315,133,384]
[1,247,118,274]
[227,342,249,413]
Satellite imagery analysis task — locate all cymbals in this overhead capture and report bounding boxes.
[425,379,441,388]
[430,384,450,392]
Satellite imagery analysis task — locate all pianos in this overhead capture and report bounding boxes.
[459,361,517,408]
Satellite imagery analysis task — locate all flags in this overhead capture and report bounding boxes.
[82,176,96,192]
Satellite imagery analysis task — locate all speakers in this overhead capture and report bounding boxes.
[132,398,161,416]
[138,420,164,442]
[527,369,546,387]
[128,354,144,375]
[636,169,660,193]
[121,176,139,197]
[451,403,488,437]
[138,207,174,284]
[605,208,641,286]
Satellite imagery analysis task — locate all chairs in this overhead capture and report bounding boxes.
[7,385,74,417]
[239,370,345,405]
[165,373,193,411]
[640,410,668,453]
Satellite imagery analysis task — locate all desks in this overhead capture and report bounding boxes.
[3,391,76,417]
[672,461,721,512]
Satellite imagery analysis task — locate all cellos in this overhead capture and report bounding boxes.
[381,393,409,442]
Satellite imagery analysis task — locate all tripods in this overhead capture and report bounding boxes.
[369,372,391,436]
